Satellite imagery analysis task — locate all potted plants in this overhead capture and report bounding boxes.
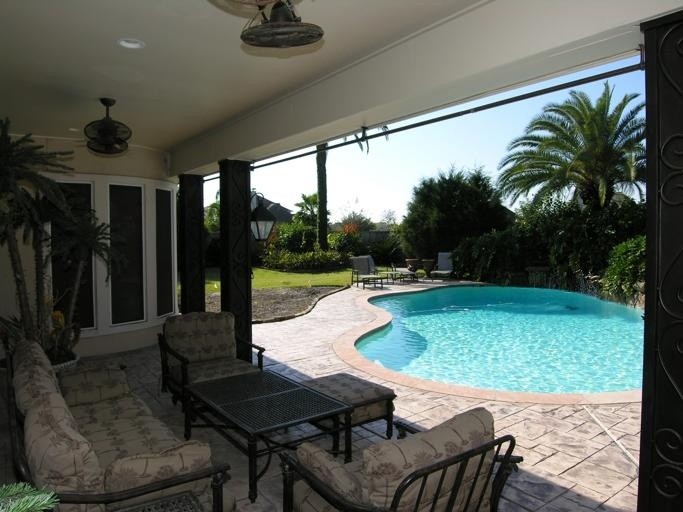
[0,111,115,375]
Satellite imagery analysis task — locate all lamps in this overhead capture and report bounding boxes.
[249,188,275,241]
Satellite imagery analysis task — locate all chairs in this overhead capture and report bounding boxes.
[348,250,459,291]
[276,399,520,510]
[156,307,268,400]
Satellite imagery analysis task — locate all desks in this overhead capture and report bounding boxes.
[174,367,355,506]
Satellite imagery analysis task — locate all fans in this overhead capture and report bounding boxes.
[234,0,326,49]
[82,96,134,144]
[84,116,128,157]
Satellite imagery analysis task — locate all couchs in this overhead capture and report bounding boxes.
[1,309,234,512]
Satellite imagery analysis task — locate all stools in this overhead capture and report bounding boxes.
[299,369,398,457]
